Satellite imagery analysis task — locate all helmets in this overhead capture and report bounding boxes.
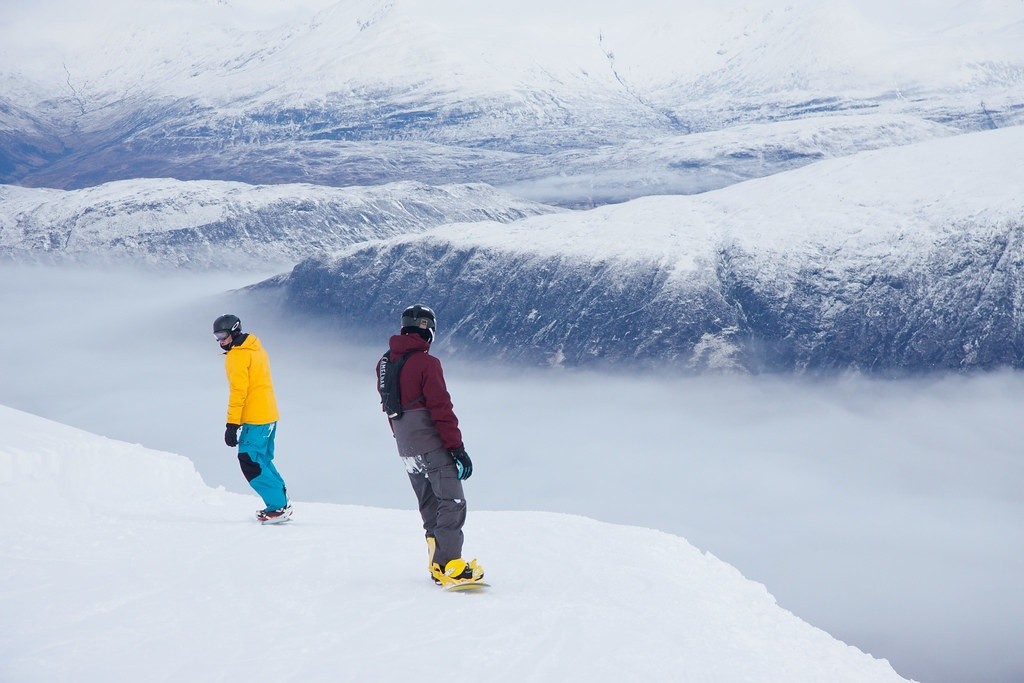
[401,302,436,331]
[213,313,242,341]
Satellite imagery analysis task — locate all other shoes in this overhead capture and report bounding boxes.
[425,536,485,588]
[254,500,293,523]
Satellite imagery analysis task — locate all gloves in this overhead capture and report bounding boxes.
[449,441,473,481]
[224,423,238,448]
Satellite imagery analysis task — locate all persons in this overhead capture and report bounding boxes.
[212,314,293,523]
[374,304,485,585]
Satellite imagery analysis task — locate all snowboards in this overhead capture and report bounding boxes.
[442,580,493,593]
[261,516,293,525]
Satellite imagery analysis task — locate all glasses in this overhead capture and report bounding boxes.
[213,330,230,340]
